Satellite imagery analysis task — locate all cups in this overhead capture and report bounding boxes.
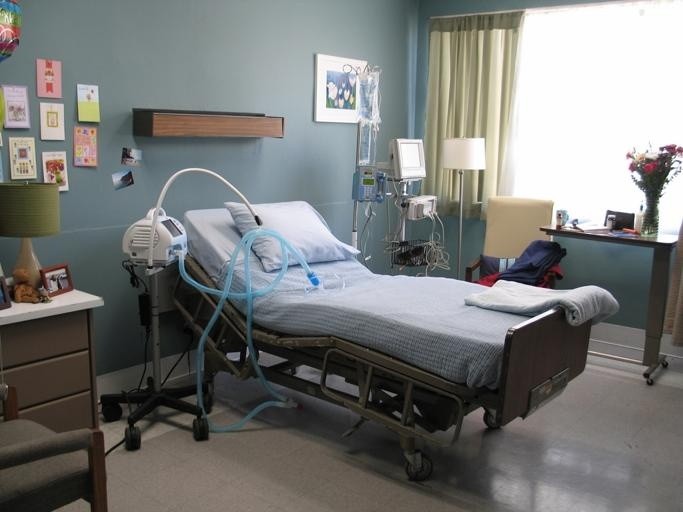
[557,210,569,226]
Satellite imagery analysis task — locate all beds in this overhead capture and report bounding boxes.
[152,200,591,481]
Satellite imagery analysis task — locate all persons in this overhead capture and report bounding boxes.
[49,275,60,292]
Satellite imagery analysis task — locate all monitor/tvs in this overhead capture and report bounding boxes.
[391,139,426,181]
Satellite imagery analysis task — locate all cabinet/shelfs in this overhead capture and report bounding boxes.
[1,287,103,436]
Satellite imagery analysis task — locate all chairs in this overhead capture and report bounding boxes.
[1,382,109,512]
[465,197,557,291]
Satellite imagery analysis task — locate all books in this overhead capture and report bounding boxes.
[577,221,609,232]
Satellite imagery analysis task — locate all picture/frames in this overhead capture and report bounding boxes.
[39,263,73,297]
[313,53,367,124]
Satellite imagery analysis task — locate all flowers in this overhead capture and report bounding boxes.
[627,145,683,232]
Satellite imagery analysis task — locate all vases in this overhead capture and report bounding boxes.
[639,194,661,239]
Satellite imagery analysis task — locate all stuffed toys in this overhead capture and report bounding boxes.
[11,269,40,303]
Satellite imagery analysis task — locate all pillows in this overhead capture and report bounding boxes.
[225,200,354,273]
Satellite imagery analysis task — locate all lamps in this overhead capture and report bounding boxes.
[446,139,487,280]
[0,182,60,294]
